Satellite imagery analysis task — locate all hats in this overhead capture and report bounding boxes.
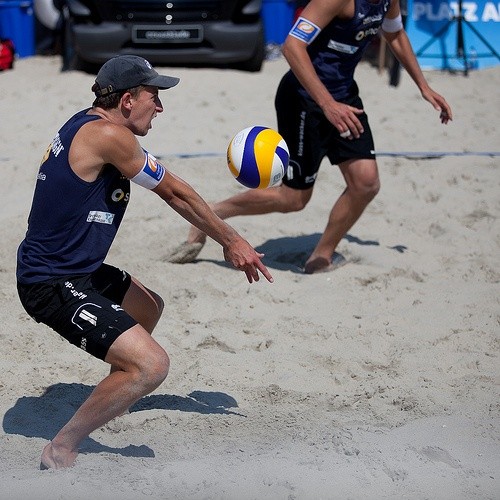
[93,55,181,97]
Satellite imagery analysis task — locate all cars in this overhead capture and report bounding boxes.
[33,0,266,73]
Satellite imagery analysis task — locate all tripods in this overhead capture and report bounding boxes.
[417,1,500,77]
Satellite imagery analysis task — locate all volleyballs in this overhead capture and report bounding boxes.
[228,127,291,189]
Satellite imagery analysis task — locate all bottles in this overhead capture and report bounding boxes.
[468,45,479,69]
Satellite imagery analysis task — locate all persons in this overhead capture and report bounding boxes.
[175,0,456,273]
[15,55,273,471]
